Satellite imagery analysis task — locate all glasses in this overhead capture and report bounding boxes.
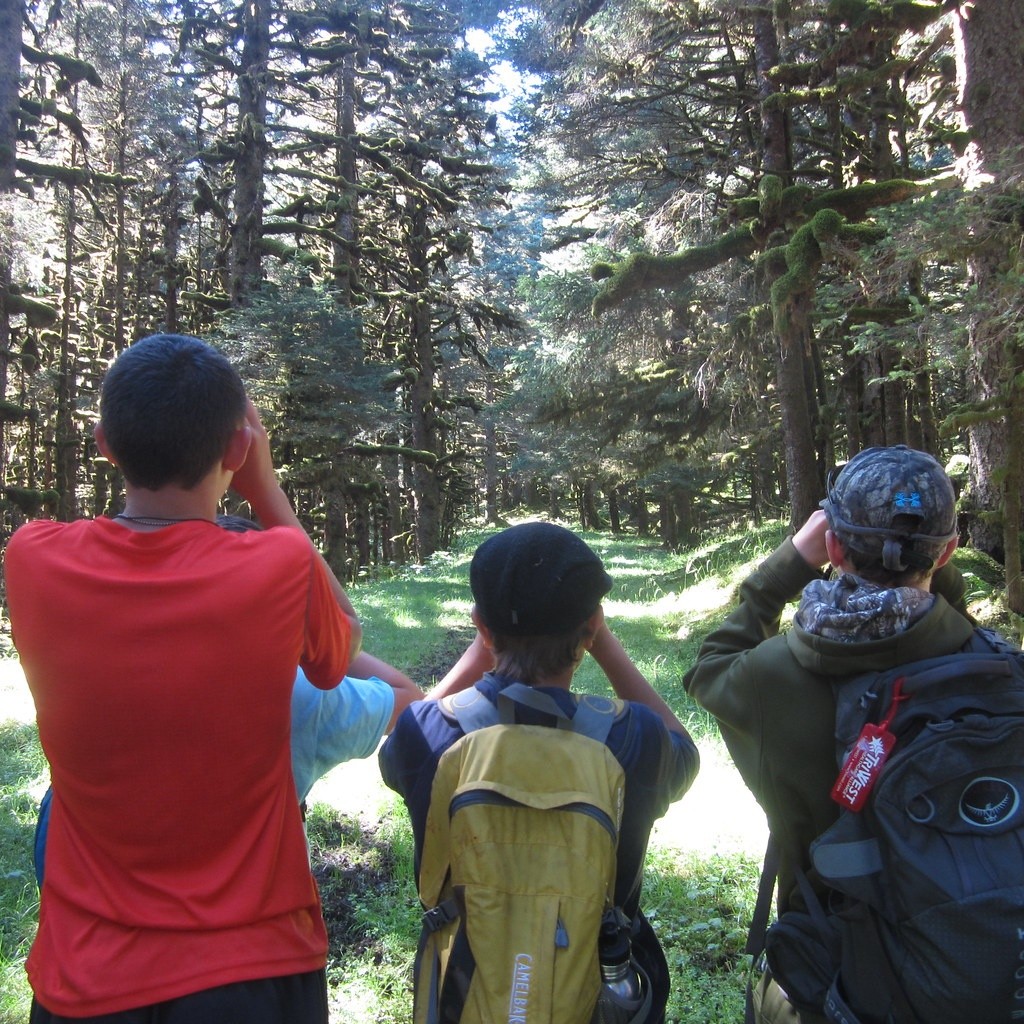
[826,463,846,506]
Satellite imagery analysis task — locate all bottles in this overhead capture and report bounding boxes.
[597,922,643,1024]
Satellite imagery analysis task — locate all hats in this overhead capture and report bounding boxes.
[818,445,960,572]
[469,521,613,630]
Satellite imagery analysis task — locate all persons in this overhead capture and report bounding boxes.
[683,443,1024,1024]
[378,522,699,1024]
[4,335,424,1024]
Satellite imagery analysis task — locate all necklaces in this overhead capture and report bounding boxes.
[118,513,175,525]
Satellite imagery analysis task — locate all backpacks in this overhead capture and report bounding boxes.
[805,628,1023,1024]
[410,682,626,1023]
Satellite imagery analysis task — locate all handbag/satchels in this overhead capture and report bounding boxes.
[764,911,859,1024]
